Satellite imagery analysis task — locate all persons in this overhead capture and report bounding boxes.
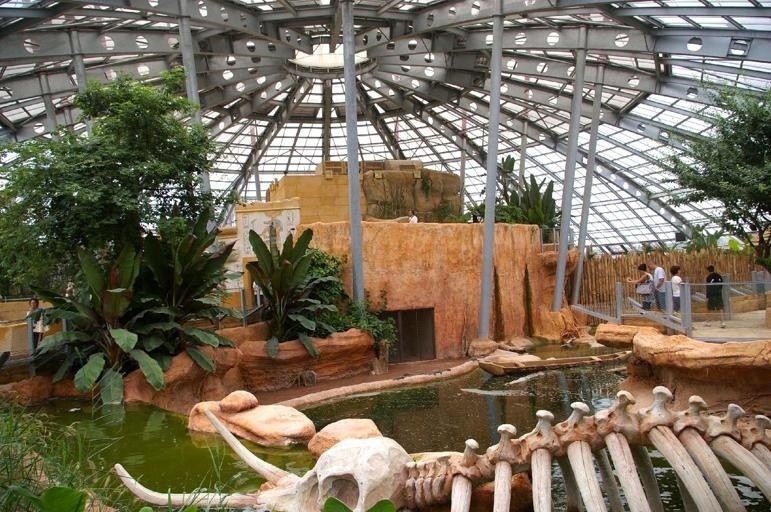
[703,266,726,328]
[26,298,49,351]
[408,210,418,223]
[648,261,666,311]
[252,281,263,306]
[670,266,683,312]
[626,264,654,311]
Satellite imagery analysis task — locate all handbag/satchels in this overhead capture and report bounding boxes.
[637,275,652,295]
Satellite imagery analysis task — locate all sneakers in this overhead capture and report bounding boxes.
[703,322,710,326]
[720,322,725,328]
